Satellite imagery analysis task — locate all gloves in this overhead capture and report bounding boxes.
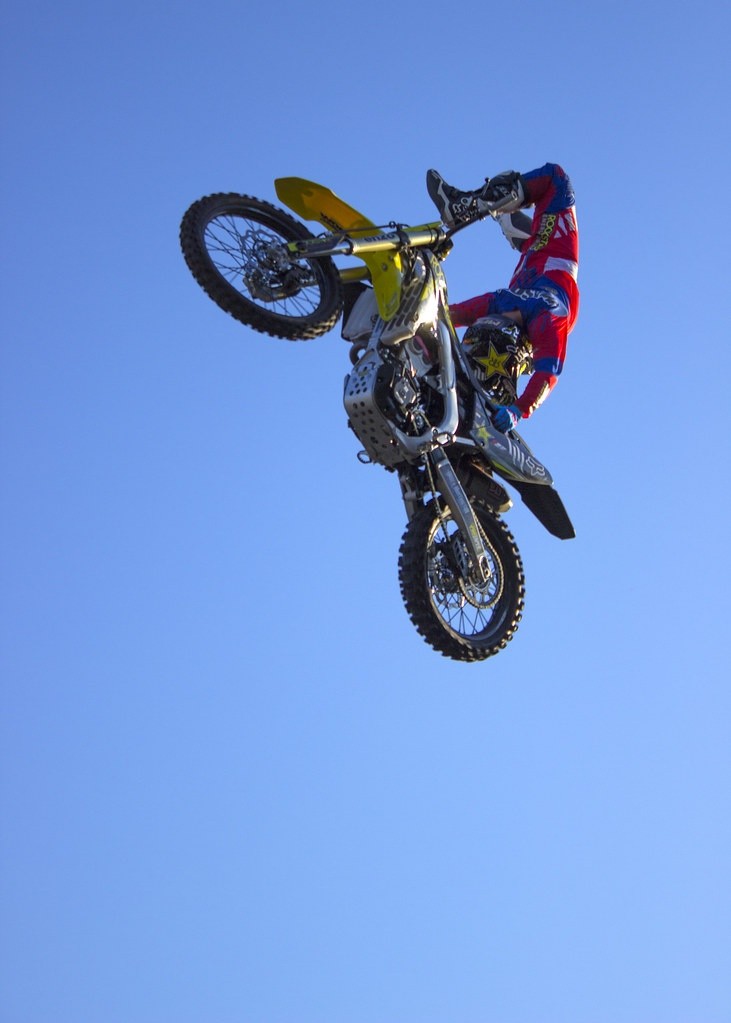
[489,404,522,434]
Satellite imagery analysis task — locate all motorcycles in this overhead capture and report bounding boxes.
[176,172,580,663]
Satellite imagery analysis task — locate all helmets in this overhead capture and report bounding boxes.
[461,315,535,391]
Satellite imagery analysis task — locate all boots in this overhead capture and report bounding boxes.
[495,210,533,253]
[426,168,530,231]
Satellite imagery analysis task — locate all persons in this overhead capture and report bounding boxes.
[426,162,579,433]
